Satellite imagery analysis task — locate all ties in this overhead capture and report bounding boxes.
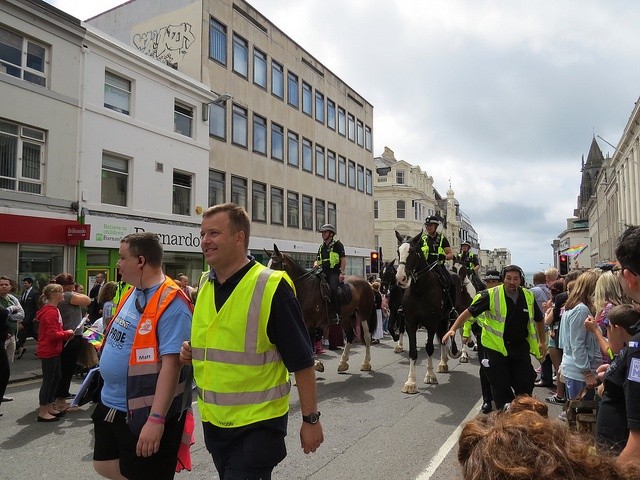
[23,290,27,305]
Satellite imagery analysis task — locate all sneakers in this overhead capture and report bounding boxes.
[16,347,27,360]
[544,396,566,405]
[64,392,77,399]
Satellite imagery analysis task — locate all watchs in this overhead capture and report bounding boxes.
[303,410,321,425]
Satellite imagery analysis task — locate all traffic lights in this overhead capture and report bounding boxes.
[568,256,571,271]
[371,252,378,273]
[559,256,568,276]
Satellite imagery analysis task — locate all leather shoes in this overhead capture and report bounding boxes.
[533,379,554,387]
[480,401,492,413]
[37,413,60,421]
[47,411,67,416]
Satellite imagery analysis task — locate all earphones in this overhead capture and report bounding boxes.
[137,256,142,265]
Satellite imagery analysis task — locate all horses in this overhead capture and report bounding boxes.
[395,228,449,393]
[264,244,376,371]
[449,255,478,362]
[379,258,405,353]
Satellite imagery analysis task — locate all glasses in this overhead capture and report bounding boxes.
[610,264,636,277]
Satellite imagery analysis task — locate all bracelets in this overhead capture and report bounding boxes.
[148,411,165,424]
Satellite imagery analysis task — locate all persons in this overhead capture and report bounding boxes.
[313,223,347,314]
[543,278,569,405]
[553,271,581,360]
[179,201,326,479]
[174,272,199,303]
[412,213,458,293]
[448,239,482,297]
[474,267,507,294]
[593,223,640,472]
[592,270,635,364]
[49,272,90,400]
[89,273,106,301]
[0,276,27,403]
[380,282,391,335]
[526,271,553,316]
[35,282,74,424]
[0,303,11,406]
[557,270,601,404]
[511,394,548,418]
[455,397,639,480]
[606,303,640,346]
[73,283,86,295]
[91,232,190,480]
[313,328,326,354]
[19,278,43,356]
[101,281,119,327]
[369,275,376,285]
[368,281,384,344]
[442,264,547,410]
[584,312,624,383]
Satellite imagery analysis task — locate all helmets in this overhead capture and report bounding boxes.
[459,241,472,248]
[317,224,336,235]
[423,215,439,225]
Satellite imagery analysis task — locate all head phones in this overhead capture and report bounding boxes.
[499,265,526,286]
